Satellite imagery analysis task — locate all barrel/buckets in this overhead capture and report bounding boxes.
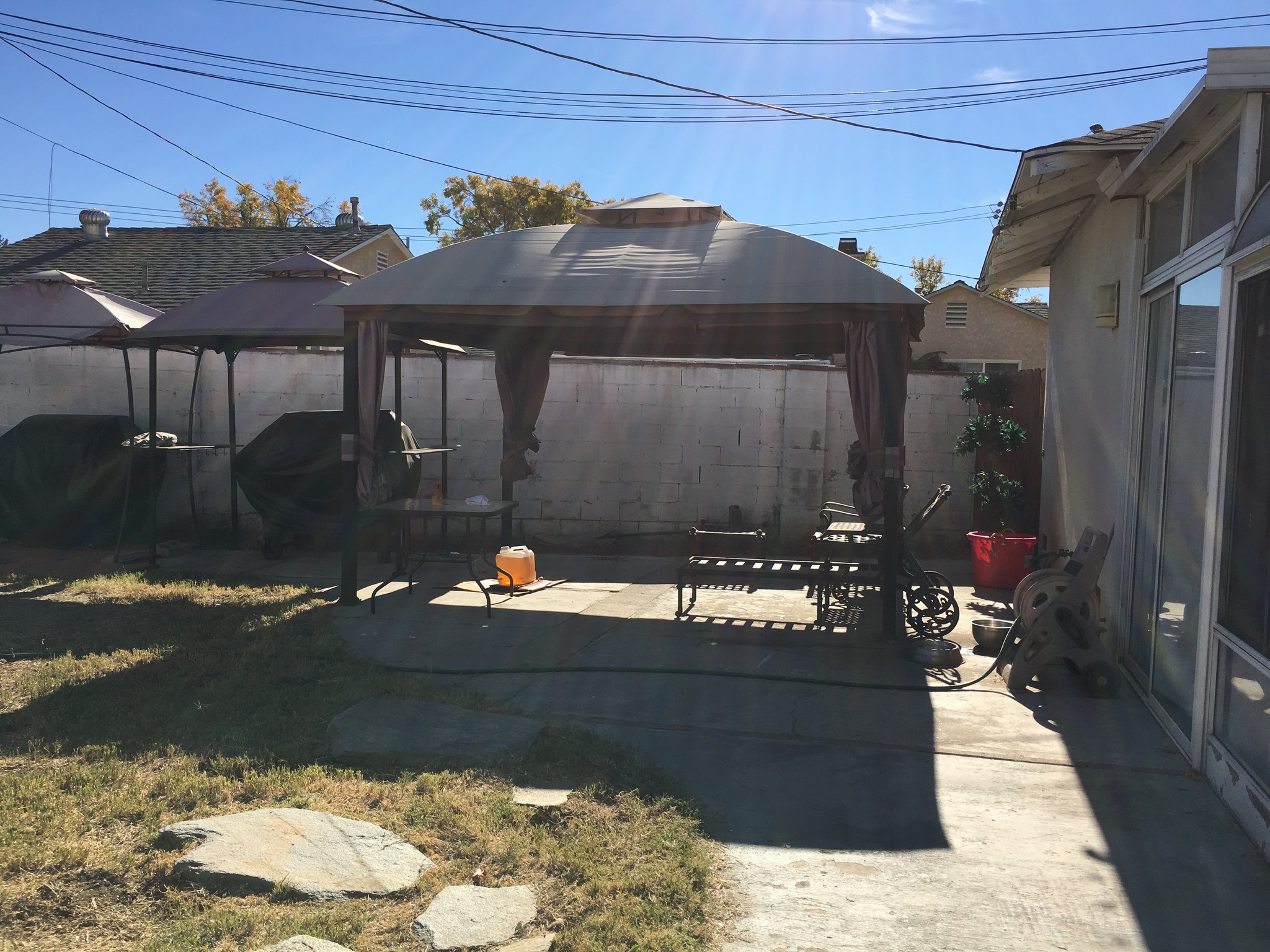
[495,545,537,586]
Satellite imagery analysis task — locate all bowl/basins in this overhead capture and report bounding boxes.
[972,618,1013,650]
[910,639,966,666]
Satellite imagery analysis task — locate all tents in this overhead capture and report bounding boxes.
[127,243,472,579]
[316,191,931,649]
[1,268,209,567]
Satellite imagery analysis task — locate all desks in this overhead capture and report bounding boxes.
[367,496,522,621]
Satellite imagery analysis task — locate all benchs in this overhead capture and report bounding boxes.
[676,551,899,634]
